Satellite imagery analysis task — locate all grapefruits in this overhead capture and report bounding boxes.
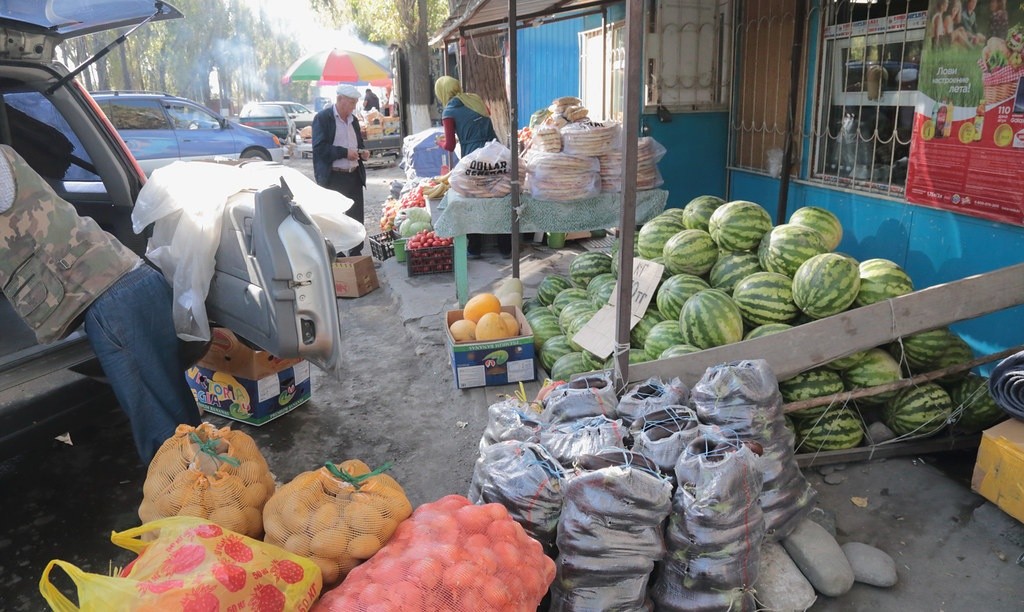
[449,292,520,343]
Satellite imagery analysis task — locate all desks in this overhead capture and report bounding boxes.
[363,135,402,150]
[435,187,669,308]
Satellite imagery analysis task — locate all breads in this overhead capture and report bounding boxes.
[546,97,588,127]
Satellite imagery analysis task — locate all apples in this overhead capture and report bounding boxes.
[399,185,426,207]
[406,228,453,272]
[381,200,399,233]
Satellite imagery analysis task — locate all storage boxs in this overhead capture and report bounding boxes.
[445,305,536,390]
[404,240,454,276]
[970,417,1024,524]
[332,256,380,298]
[184,327,312,425]
[369,230,402,262]
[363,111,402,140]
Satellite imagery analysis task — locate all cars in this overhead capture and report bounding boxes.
[244,104,297,147]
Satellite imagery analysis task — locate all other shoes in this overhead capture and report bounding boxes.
[467,251,481,259]
[501,252,512,259]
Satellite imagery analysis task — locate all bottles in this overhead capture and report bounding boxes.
[935,98,947,138]
[943,98,953,137]
[931,100,939,123]
[973,99,985,142]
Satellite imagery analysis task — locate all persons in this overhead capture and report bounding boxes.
[363,89,379,111]
[930,0,1007,52]
[311,84,381,269]
[434,76,501,159]
[0,144,204,470]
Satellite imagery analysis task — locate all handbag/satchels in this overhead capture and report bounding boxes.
[448,138,525,199]
[38,516,324,612]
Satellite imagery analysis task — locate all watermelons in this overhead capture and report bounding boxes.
[522,193,1003,455]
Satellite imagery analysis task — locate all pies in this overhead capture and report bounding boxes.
[451,125,660,201]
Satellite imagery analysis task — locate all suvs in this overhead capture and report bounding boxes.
[86,90,285,182]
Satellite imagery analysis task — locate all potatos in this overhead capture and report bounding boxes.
[139,429,557,612]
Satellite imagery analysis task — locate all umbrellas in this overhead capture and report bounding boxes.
[281,47,395,87]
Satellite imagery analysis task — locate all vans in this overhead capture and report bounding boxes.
[238,101,319,128]
[0,0,186,460]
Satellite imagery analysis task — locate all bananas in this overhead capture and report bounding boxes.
[422,170,453,200]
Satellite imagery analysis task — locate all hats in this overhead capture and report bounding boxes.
[336,85,360,98]
